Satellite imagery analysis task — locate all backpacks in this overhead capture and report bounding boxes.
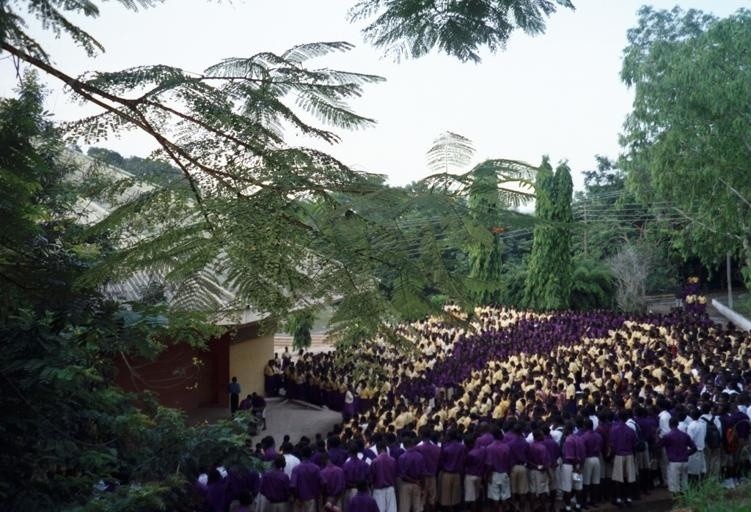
[702,415,721,450]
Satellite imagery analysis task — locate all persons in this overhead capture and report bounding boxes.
[186,302,749,512]
[675,276,707,307]
